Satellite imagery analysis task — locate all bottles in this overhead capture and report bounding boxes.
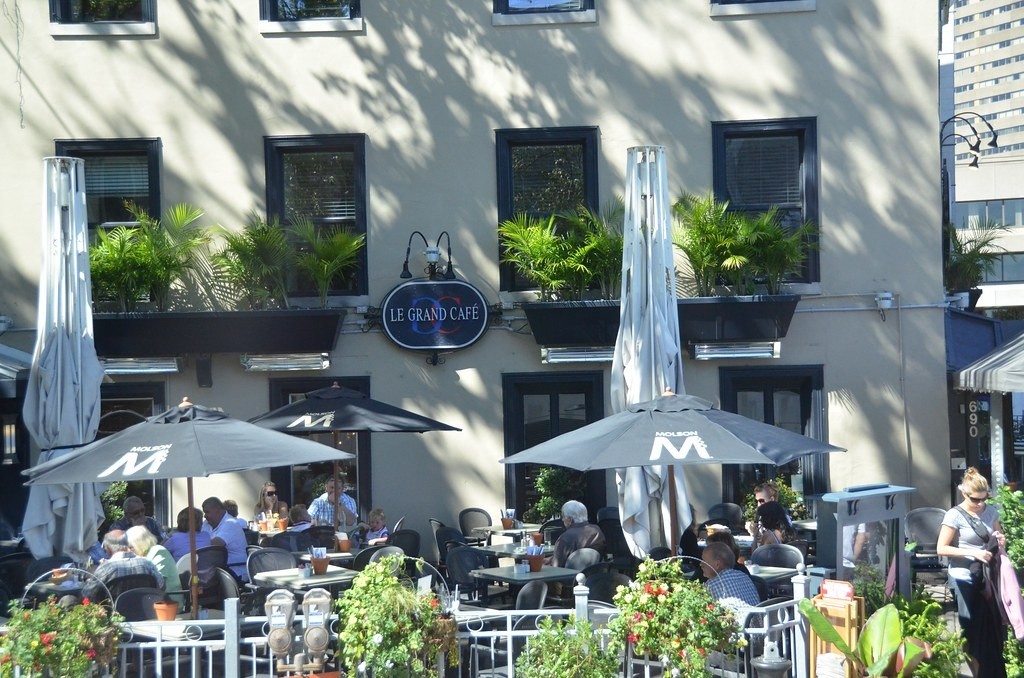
[522,559,531,573]
[521,534,535,551]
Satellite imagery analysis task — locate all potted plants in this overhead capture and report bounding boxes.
[944,216,1017,311]
[494,190,823,346]
[89,197,368,358]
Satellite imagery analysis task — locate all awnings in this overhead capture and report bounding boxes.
[952,329,1024,395]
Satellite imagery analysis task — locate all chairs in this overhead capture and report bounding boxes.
[904,506,956,602]
[21,506,805,678]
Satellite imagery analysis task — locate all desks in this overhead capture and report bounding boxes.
[253,564,361,601]
[434,602,505,678]
[24,579,87,602]
[697,535,755,558]
[745,565,799,602]
[115,608,250,678]
[290,549,354,567]
[475,541,555,609]
[790,518,818,566]
[467,563,581,609]
[473,522,543,564]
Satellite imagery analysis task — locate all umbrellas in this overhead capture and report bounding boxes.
[245,381,464,551]
[611,160,693,562]
[498,386,849,564]
[20,171,107,560]
[20,396,356,621]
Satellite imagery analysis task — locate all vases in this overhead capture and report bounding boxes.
[83,625,116,650]
[428,613,455,638]
[530,533,544,547]
[153,600,179,621]
[277,518,289,532]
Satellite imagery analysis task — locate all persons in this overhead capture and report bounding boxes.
[253,481,289,519]
[358,508,389,546]
[937,467,1008,678]
[680,503,764,641]
[852,521,888,567]
[82,496,250,614]
[288,504,313,532]
[749,482,800,555]
[308,474,359,526]
[543,500,606,568]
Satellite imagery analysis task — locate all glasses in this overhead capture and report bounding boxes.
[264,490,279,496]
[126,508,145,515]
[754,498,771,503]
[967,493,987,502]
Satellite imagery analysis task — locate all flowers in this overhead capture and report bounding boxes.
[608,556,749,678]
[335,553,460,678]
[0,593,127,678]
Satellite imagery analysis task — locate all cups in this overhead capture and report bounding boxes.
[530,533,543,547]
[501,518,513,529]
[258,517,288,532]
[524,554,545,572]
[338,540,352,551]
[310,556,331,576]
[526,545,543,555]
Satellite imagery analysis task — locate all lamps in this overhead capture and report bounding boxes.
[238,351,329,371]
[689,341,781,360]
[939,117,981,155]
[940,112,999,148]
[541,346,615,364]
[874,292,895,309]
[98,357,183,375]
[945,292,970,311]
[939,134,979,171]
[399,231,428,279]
[435,231,456,279]
